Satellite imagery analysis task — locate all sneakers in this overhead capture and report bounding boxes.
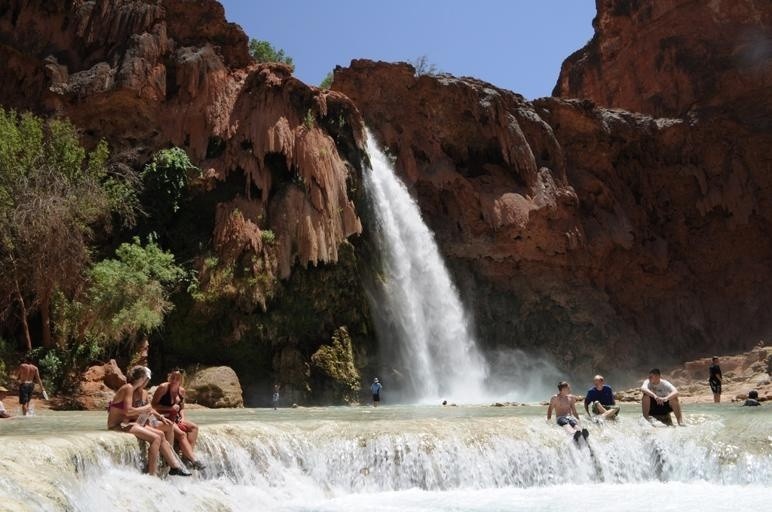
[169,467,192,476]
[193,461,206,470]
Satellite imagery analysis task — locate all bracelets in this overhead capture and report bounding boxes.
[663,398,668,403]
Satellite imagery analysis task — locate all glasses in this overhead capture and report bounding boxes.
[173,367,185,374]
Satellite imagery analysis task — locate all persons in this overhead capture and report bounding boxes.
[15,358,45,415]
[107,365,207,476]
[370,377,383,407]
[547,382,589,442]
[709,358,723,402]
[272,384,281,410]
[584,375,620,423]
[0,386,11,418]
[640,368,685,425]
[743,391,761,406]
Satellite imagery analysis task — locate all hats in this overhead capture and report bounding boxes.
[373,378,379,383]
